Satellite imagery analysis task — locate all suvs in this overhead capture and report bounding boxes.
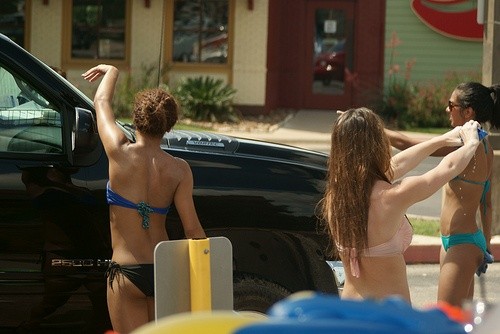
[0,31,343,333]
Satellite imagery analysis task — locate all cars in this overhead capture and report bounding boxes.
[172,24,229,66]
[313,37,347,87]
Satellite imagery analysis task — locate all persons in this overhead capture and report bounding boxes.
[313,107,481,309]
[81,64,206,334]
[336,81,500,310]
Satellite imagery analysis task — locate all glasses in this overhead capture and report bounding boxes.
[448,100,465,111]
